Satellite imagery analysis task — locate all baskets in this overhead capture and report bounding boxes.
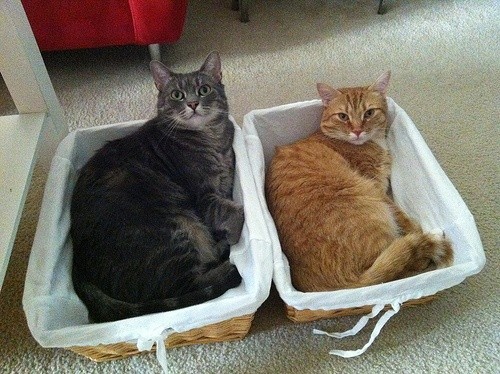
[242,93,486,323]
[22,115,273,361]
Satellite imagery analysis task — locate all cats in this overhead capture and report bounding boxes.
[69,50,245,324]
[264,69,455,293]
